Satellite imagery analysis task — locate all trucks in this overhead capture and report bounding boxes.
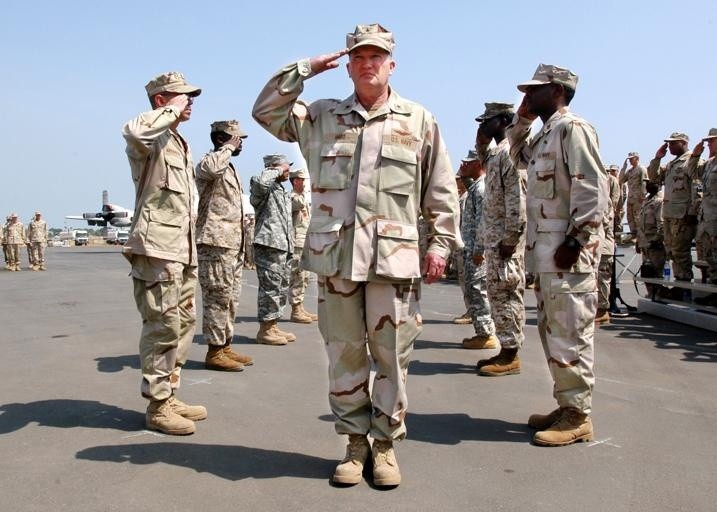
[105,230,128,244]
[55,230,87,247]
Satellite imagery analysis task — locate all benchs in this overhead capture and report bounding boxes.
[632,260,716,337]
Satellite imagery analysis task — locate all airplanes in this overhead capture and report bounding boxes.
[64,190,137,227]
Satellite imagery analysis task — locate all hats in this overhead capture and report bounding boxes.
[6,211,41,220]
[289,170,307,178]
[262,154,294,167]
[146,72,202,97]
[346,24,395,56]
[606,127,717,182]
[210,121,248,139]
[462,63,578,162]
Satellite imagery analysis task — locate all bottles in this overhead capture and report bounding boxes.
[663,261,669,280]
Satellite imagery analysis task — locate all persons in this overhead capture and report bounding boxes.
[455,147,500,352]
[249,154,300,345]
[473,101,529,377]
[595,127,717,321]
[195,120,254,373]
[290,168,323,323]
[248,22,468,490]
[504,63,612,451]
[242,212,257,270]
[1,211,50,272]
[121,70,209,435]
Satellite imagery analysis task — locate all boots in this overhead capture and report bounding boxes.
[145,398,206,439]
[464,335,497,349]
[528,405,566,429]
[299,301,318,320]
[332,433,371,485]
[4,262,46,271]
[476,347,521,377]
[271,322,297,342]
[223,341,254,365]
[454,311,474,324]
[204,345,245,372]
[372,438,400,486]
[532,410,594,445]
[290,304,312,322]
[255,323,286,344]
